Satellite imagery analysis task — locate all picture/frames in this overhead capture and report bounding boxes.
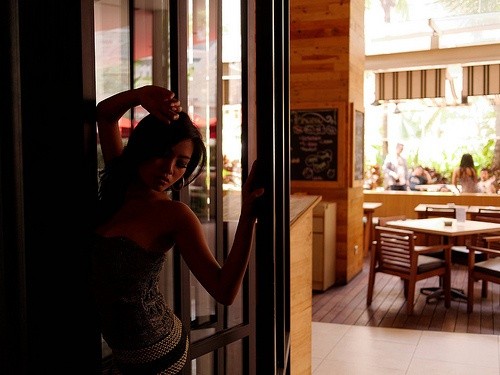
[350,102,365,190]
[290,101,347,190]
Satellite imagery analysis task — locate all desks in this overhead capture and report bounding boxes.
[414,203,468,247]
[465,206,500,222]
[386,216,500,310]
[363,202,383,260]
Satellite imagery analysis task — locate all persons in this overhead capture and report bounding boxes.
[89,85,265,375]
[453,154,500,194]
[369,143,450,192]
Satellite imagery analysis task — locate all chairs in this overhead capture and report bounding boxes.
[362,206,500,315]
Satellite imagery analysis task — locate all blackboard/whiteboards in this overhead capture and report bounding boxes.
[289,101,349,189]
[350,100,365,188]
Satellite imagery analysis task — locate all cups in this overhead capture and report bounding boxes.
[455,207,466,226]
